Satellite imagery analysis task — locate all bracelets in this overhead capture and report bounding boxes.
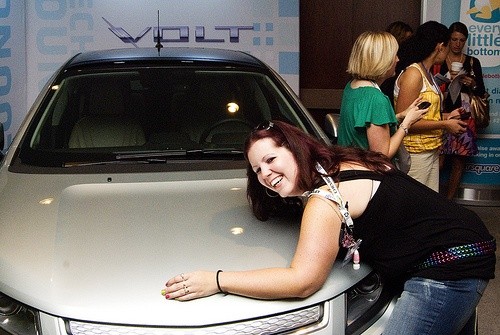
[471,84,478,89]
[216,270,228,296]
[399,123,408,137]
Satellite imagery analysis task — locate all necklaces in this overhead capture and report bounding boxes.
[448,53,464,64]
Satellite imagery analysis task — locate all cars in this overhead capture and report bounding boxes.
[0,47,397,335]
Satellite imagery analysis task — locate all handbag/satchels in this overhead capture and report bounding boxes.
[460,57,491,127]
[391,140,411,174]
[440,127,479,158]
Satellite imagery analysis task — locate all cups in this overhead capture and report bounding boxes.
[451,61,463,72]
[464,188,475,200]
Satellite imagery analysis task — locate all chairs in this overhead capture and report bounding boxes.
[188,80,249,145]
[68,81,145,148]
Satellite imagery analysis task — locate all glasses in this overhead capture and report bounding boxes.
[252,120,275,131]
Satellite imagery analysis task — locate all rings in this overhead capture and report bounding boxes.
[182,281,187,289]
[464,82,466,85]
[180,273,185,282]
[184,288,190,295]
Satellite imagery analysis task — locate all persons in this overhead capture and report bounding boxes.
[162,121,496,335]
[336,21,489,201]
